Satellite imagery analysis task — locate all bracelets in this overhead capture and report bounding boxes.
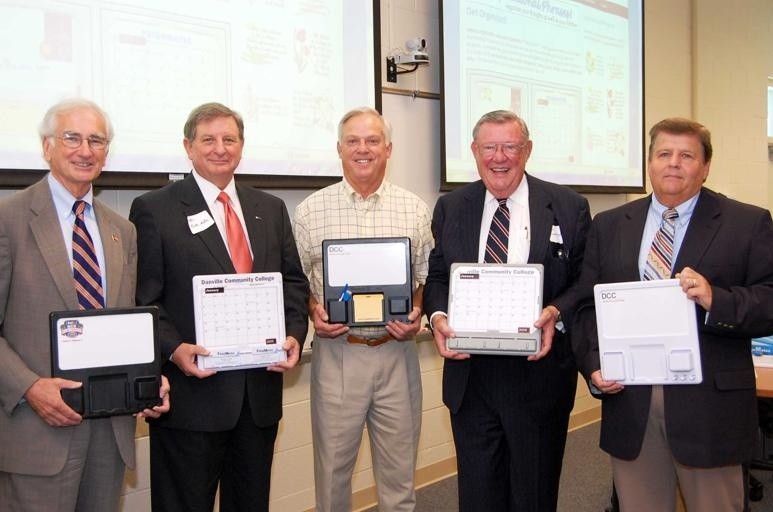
[546,305,561,321]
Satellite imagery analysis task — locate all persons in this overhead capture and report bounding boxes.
[421,110,591,511]
[290,108,435,512]
[127,103,310,512]
[1,99,170,512]
[565,118,773,511]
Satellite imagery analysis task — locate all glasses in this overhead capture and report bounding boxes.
[49,133,108,150]
[474,142,528,156]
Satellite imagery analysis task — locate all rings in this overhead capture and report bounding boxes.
[693,279,696,287]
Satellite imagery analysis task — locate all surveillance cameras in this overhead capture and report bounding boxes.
[407,37,426,50]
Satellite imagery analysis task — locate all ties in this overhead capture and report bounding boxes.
[66,200,108,312]
[642,206,682,283]
[215,189,255,275]
[481,194,511,265]
